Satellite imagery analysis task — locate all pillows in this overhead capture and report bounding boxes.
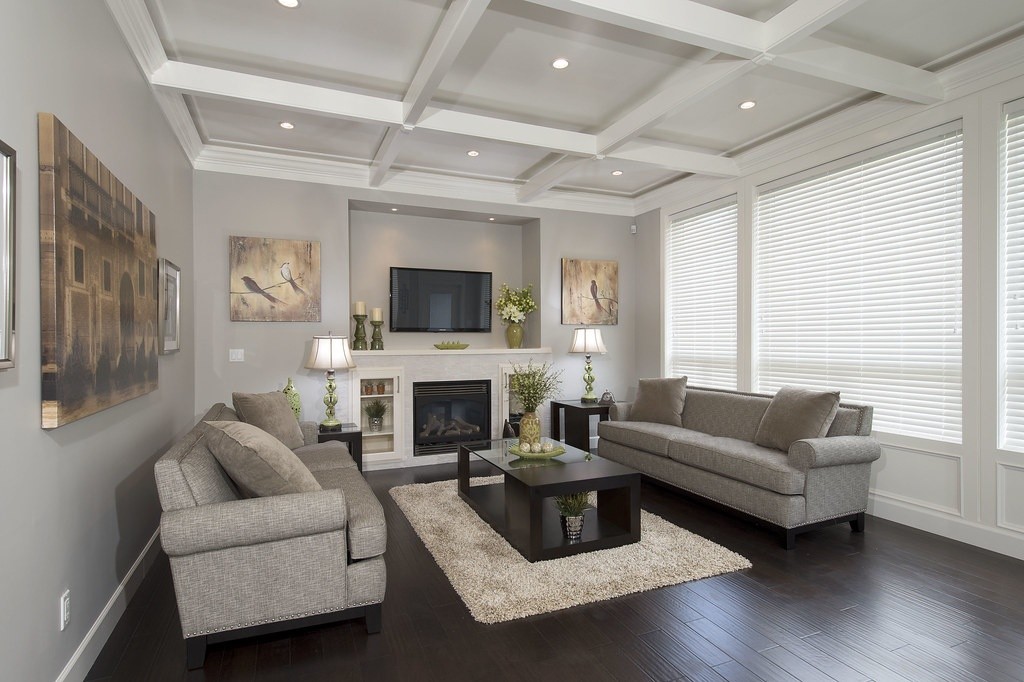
[203,390,322,498]
[626,376,841,452]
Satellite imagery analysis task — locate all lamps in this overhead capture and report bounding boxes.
[303,329,356,432]
[568,328,607,403]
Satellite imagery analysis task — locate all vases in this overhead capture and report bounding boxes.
[282,377,301,423]
[507,321,524,349]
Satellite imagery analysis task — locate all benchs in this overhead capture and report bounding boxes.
[597,388,881,550]
[153,403,387,672]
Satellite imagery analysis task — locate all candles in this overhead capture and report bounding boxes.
[372,308,383,321]
[355,302,366,315]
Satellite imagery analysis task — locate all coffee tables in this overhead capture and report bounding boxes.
[457,436,642,564]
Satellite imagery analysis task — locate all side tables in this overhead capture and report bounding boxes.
[318,423,362,476]
[550,400,634,453]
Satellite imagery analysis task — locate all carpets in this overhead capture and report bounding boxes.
[388,474,753,625]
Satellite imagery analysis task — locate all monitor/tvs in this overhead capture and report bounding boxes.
[389,267,492,333]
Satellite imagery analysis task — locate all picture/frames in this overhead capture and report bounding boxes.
[0,139,16,369]
[158,257,181,356]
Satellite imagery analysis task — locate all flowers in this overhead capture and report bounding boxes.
[493,280,537,327]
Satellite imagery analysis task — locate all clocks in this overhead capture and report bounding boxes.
[598,389,615,405]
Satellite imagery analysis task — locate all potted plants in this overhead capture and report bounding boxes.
[551,491,597,539]
[504,356,567,445]
[362,379,391,432]
